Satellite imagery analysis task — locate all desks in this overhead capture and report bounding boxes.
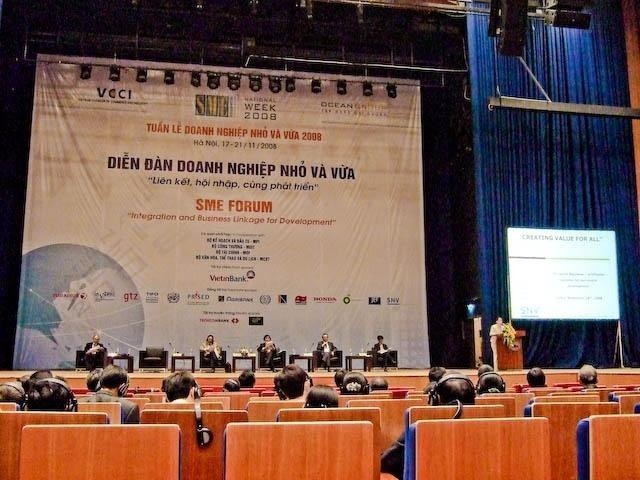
[107,356,133,373]
[345,355,371,372]
[233,356,255,374]
[171,356,195,374]
[289,355,315,372]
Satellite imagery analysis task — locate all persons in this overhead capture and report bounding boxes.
[239,370,256,388]
[0,382,26,403]
[317,332,338,373]
[26,379,77,412]
[526,368,546,388]
[257,334,280,373]
[489,317,505,372]
[224,378,241,394]
[18,370,67,390]
[370,377,390,390]
[80,369,106,405]
[84,334,107,371]
[373,336,398,373]
[274,365,313,400]
[199,334,222,373]
[335,370,349,388]
[85,364,140,424]
[478,364,494,377]
[429,368,448,385]
[304,384,338,408]
[380,374,476,480]
[162,372,202,405]
[578,364,598,386]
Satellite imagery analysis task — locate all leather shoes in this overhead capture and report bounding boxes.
[392,360,396,364]
[217,361,221,364]
[211,368,216,373]
[274,368,276,371]
[327,367,331,372]
[384,367,388,371]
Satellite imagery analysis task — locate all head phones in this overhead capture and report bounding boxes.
[194,400,213,446]
[99,365,129,397]
[1,382,26,401]
[165,372,202,402]
[19,379,78,411]
[427,374,477,405]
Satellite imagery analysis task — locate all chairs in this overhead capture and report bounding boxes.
[226,421,373,480]
[138,346,168,372]
[368,351,398,372]
[140,410,247,480]
[144,403,222,410]
[348,398,423,456]
[72,403,120,424]
[258,350,286,372]
[415,417,552,480]
[19,425,180,480]
[74,350,104,370]
[532,401,619,480]
[204,393,256,407]
[618,395,640,414]
[275,406,381,480]
[72,378,633,396]
[577,414,640,480]
[313,351,342,372]
[403,404,507,480]
[199,350,226,372]
[474,398,517,415]
[247,402,314,422]
[476,392,533,414]
[0,412,109,480]
